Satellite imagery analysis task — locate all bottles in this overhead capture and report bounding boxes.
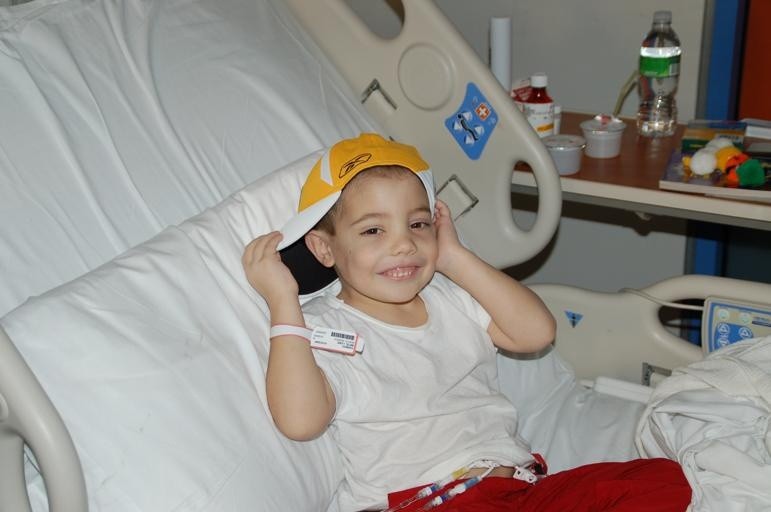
[636,10,684,137]
[520,73,556,137]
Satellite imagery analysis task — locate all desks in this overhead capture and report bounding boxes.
[497,110,770,236]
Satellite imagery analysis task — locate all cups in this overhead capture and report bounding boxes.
[540,115,627,178]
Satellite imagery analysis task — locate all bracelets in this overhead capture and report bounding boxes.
[269,324,313,343]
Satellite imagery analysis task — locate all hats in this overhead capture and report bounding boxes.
[273,133,436,294]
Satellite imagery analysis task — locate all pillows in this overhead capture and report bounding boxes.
[3,145,353,512]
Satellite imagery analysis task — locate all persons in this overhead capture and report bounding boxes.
[241,132,692,512]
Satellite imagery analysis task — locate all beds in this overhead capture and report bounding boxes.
[3,1,771,512]
